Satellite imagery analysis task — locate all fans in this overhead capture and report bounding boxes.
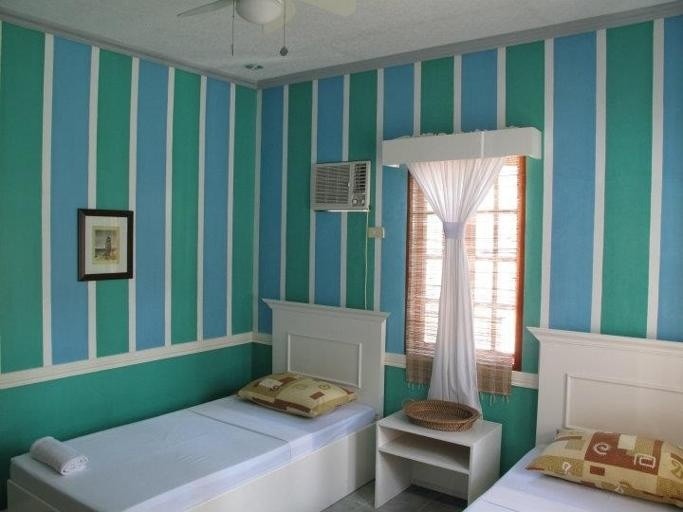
[176,0,358,35]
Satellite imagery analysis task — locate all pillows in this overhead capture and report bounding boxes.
[525,424,682,508]
[236,371,356,419]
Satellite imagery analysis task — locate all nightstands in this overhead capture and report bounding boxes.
[375,406,503,509]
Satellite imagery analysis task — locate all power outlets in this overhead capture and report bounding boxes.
[368,228,385,239]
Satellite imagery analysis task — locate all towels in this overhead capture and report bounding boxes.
[30,435,90,476]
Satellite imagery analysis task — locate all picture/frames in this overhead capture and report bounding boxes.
[76,207,134,282]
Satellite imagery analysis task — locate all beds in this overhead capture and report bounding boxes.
[6,297,392,511]
[459,326,682,511]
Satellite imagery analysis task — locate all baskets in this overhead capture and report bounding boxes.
[396,395,484,432]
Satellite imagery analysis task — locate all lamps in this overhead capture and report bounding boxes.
[230,1,287,58]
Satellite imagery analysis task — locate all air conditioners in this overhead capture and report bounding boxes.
[311,160,371,211]
